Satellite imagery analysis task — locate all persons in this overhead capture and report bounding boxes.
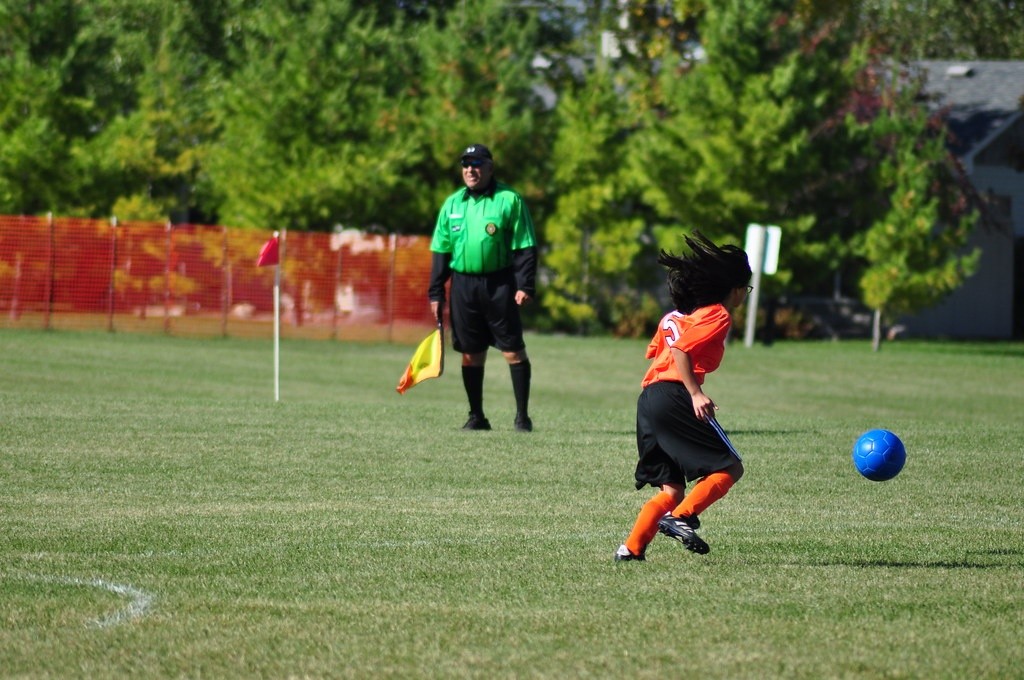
[429,143,537,431]
[613,228,753,563]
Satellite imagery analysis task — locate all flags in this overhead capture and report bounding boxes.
[256,234,281,266]
[396,329,441,396]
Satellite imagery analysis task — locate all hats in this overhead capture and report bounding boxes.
[459,144,492,160]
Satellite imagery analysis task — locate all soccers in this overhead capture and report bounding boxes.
[851,429,906,481]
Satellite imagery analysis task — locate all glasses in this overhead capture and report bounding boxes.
[461,159,490,168]
[738,285,753,293]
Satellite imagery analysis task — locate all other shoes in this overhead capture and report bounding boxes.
[514,413,532,432]
[462,410,491,430]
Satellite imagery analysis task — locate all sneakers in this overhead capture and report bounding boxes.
[657,511,710,555]
[614,545,646,562]
